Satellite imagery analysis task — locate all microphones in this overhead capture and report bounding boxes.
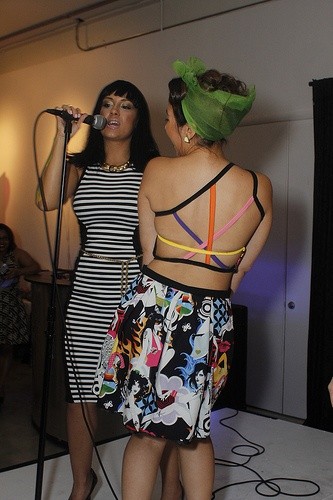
[47,108,106,131]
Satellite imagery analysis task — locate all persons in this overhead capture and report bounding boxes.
[0,223,38,403]
[92,57,281,499]
[32,79,163,499]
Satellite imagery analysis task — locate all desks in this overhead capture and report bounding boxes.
[24,269,137,445]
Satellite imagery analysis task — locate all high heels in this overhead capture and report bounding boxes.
[66,468,98,500]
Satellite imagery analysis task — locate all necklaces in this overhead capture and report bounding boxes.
[99,158,133,174]
